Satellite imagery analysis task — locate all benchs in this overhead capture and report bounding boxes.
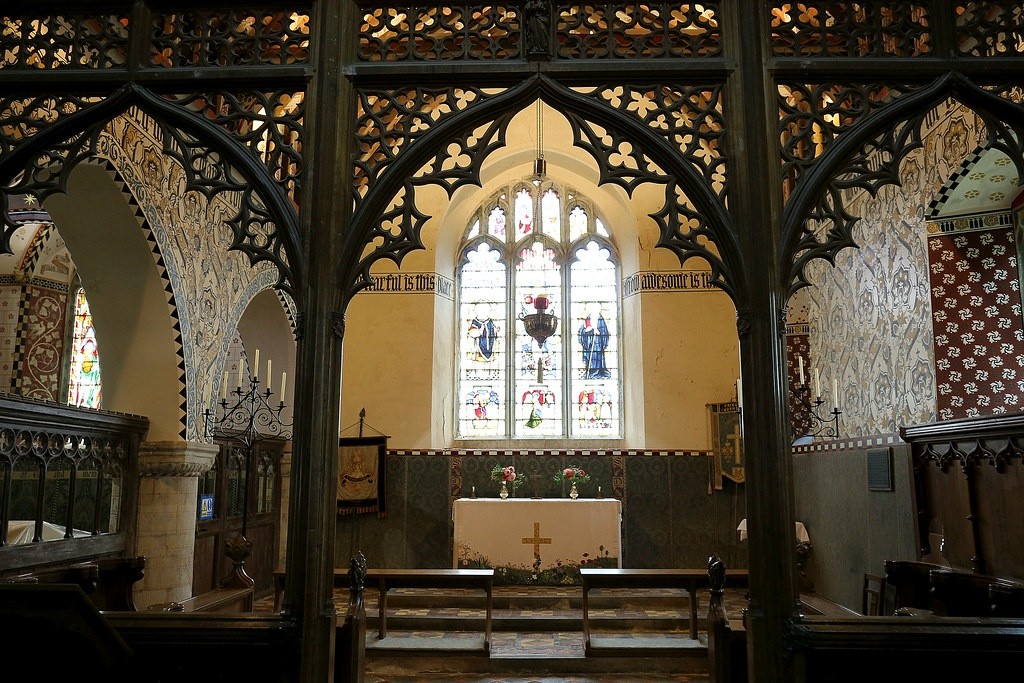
[98,549,367,683]
[706,551,936,683]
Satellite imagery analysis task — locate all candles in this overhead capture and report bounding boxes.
[814,368,821,397]
[736,379,741,406]
[799,356,804,385]
[221,371,228,399]
[254,348,259,377]
[237,358,243,387]
[280,372,286,401]
[206,380,211,409]
[267,359,272,388]
[832,379,838,407]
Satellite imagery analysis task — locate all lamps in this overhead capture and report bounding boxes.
[524,97,558,384]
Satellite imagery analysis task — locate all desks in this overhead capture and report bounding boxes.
[736,518,816,601]
[452,497,625,588]
[272,566,494,658]
[578,568,750,657]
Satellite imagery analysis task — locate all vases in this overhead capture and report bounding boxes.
[569,482,579,500]
[500,485,509,500]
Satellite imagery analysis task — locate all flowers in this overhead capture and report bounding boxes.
[552,464,591,486]
[490,464,525,489]
